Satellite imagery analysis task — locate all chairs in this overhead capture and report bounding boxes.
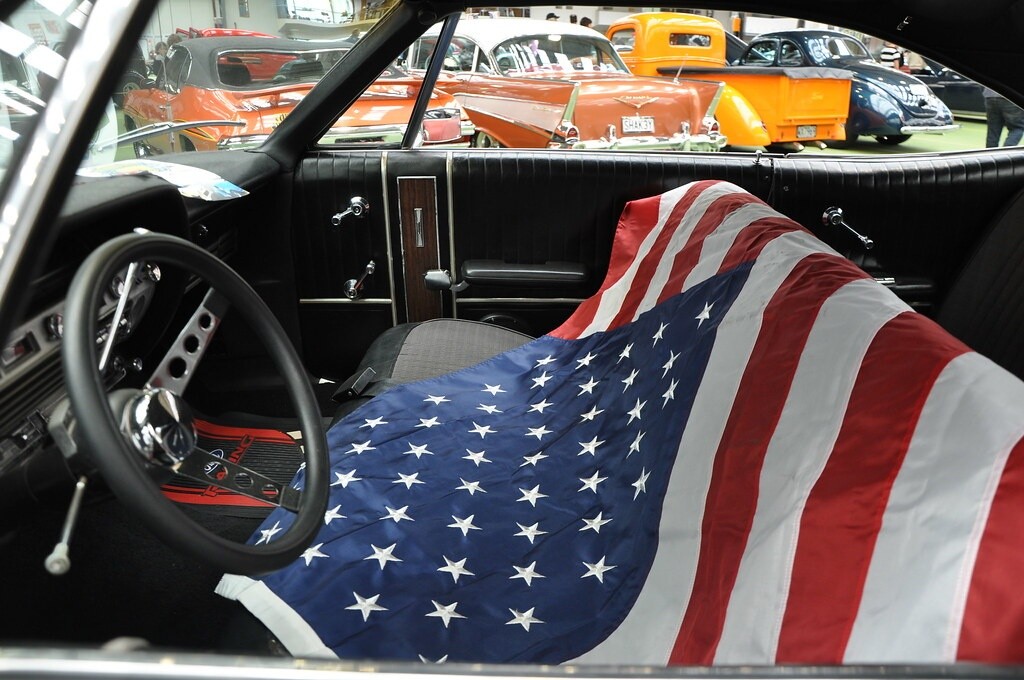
[215,61,253,88]
[280,59,326,82]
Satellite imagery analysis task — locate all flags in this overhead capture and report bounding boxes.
[215,180,1024,665]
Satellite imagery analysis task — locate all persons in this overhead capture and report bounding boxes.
[153,34,181,74]
[347,28,360,44]
[545,12,561,21]
[580,17,592,29]
[879,42,901,71]
[981,86,1024,148]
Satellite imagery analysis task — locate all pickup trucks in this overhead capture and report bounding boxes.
[568,9,854,153]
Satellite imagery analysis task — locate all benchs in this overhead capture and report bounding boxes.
[221,176,1023,679]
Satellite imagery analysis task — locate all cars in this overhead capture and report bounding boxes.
[911,56,989,124]
[123,36,476,163]
[111,39,152,108]
[400,17,730,154]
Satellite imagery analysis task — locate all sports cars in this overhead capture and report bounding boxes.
[738,29,962,151]
